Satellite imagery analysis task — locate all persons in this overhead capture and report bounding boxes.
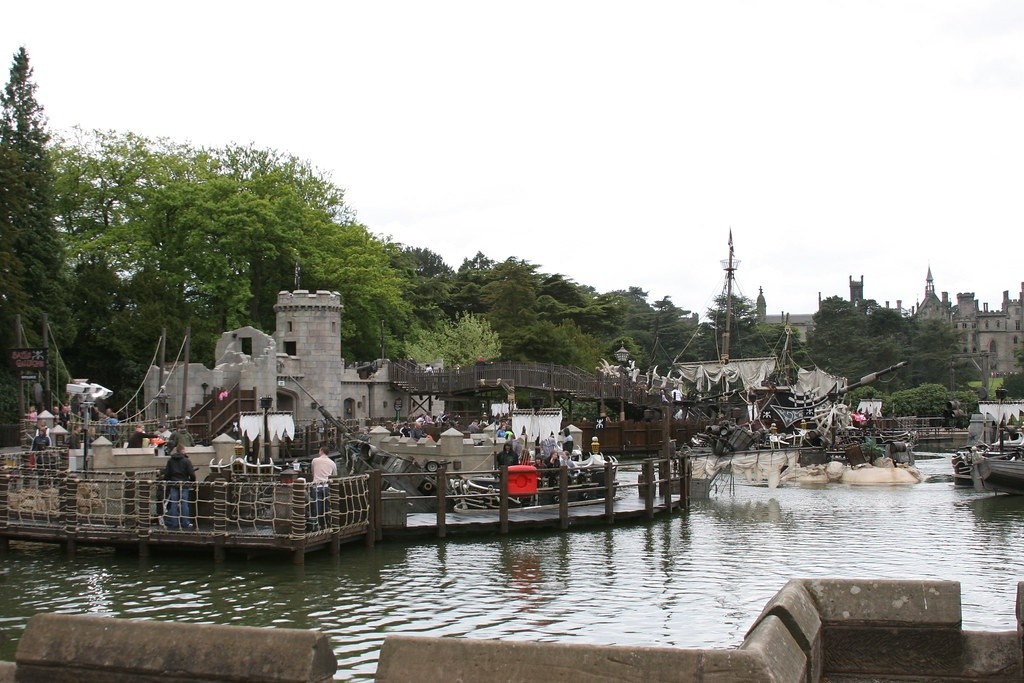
[392,357,574,491]
[27,386,229,528]
[311,446,338,528]
[851,407,869,424]
[595,367,683,420]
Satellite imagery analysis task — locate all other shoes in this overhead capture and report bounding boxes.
[186,524,193,528]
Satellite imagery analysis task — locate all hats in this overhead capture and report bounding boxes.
[158,424,165,428]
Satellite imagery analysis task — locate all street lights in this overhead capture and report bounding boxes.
[613,340,629,422]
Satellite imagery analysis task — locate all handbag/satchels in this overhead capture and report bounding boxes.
[30,453,35,468]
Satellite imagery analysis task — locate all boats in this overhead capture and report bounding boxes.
[952,383,1024,495]
[452,407,620,514]
